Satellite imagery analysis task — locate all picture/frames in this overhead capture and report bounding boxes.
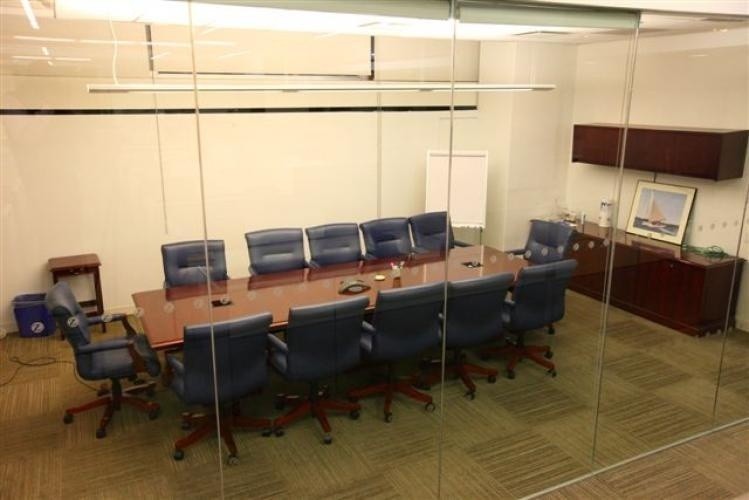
[622,179,698,246]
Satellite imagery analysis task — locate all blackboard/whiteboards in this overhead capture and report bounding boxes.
[425,149,487,229]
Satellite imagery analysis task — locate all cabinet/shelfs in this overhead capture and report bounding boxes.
[560,218,748,339]
[571,123,749,183]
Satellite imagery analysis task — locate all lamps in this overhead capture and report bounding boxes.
[82,20,559,95]
[52,1,643,42]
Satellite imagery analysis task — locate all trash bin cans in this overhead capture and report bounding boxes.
[10,292,56,338]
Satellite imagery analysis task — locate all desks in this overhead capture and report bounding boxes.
[130,243,541,353]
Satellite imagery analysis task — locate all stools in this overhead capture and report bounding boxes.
[47,253,106,341]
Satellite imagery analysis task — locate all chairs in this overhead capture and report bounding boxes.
[44,281,162,437]
[359,217,412,262]
[161,237,230,289]
[244,226,310,280]
[482,259,579,380]
[268,296,370,444]
[347,281,447,423]
[524,218,576,268]
[428,273,517,401]
[305,220,364,270]
[166,311,274,467]
[408,213,456,251]
